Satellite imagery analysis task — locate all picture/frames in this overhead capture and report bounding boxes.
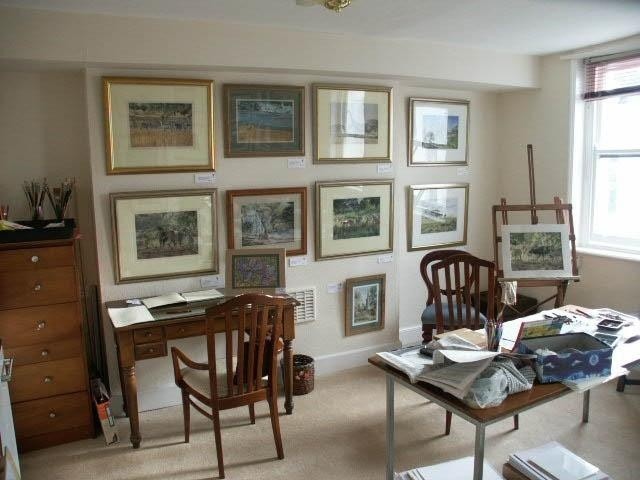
[501,224,575,278]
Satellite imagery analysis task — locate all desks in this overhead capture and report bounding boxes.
[107,284,300,451]
[362,302,640,479]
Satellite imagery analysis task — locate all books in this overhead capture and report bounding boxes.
[375,328,541,400]
[394,442,614,480]
[104,287,226,327]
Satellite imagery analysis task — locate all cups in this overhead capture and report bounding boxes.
[30,207,43,224]
[0,204,10,220]
[485,325,502,351]
[53,207,69,220]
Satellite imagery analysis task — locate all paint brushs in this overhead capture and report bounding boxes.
[23,178,48,218]
[48,176,76,218]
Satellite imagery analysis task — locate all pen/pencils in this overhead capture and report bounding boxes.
[166,310,191,314]
[564,309,585,316]
[486,318,503,352]
[0,204,9,220]
[576,309,591,318]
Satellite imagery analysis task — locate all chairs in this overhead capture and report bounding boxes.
[170,293,298,480]
[420,249,520,435]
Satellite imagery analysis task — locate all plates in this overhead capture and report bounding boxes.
[598,319,625,332]
[593,333,620,348]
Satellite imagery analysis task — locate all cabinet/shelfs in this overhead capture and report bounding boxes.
[0,227,97,456]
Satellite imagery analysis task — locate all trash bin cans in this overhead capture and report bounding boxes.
[280,354,314,395]
